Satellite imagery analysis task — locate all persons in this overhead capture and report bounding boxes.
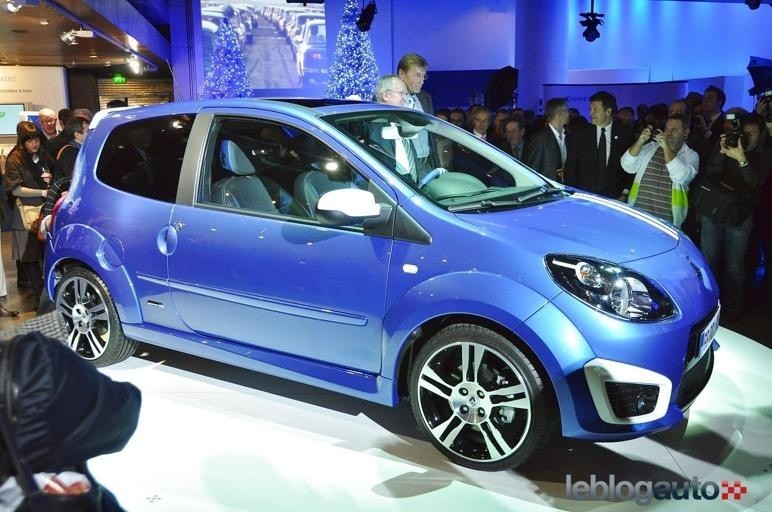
[0,330,142,512]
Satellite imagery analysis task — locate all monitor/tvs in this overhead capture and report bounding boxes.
[0,103,24,138]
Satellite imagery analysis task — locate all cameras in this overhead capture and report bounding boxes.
[724,113,748,151]
[649,127,660,136]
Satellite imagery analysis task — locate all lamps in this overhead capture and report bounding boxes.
[579,0,605,42]
[356,0,378,32]
[7,0,157,74]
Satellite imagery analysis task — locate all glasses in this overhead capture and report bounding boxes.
[405,72,429,82]
[385,89,409,98]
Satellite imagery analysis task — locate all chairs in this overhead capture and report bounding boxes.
[294,135,360,219]
[212,139,309,218]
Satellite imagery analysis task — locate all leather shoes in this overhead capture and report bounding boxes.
[0,302,19,318]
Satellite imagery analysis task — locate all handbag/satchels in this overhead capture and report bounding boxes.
[18,201,47,234]
[685,176,755,228]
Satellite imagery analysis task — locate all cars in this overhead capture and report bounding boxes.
[40,92,727,476]
[199,2,326,88]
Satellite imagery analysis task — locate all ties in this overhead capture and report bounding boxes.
[597,127,608,171]
[394,122,418,184]
[512,146,521,157]
[480,136,486,141]
[408,96,416,109]
